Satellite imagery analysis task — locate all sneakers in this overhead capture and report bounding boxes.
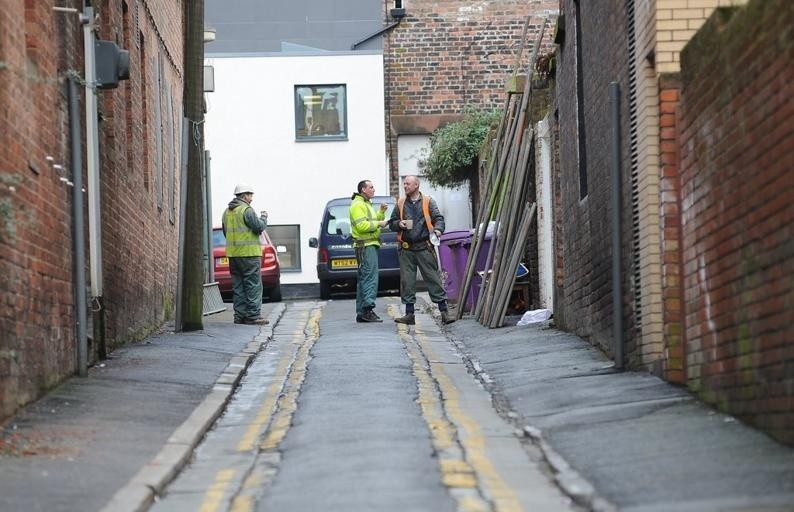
[394,313,415,326]
[356,310,383,323]
[441,311,455,325]
[235,317,271,325]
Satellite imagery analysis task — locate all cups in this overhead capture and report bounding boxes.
[404,220,413,230]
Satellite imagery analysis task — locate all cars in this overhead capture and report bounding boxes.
[212,221,283,302]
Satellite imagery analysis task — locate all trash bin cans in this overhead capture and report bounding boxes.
[437,230,499,315]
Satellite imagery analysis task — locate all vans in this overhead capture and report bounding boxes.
[306,194,401,302]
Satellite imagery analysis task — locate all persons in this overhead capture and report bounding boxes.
[386,176,455,325]
[349,180,389,323]
[222,184,270,324]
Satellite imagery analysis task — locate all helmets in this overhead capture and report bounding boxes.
[233,182,255,195]
